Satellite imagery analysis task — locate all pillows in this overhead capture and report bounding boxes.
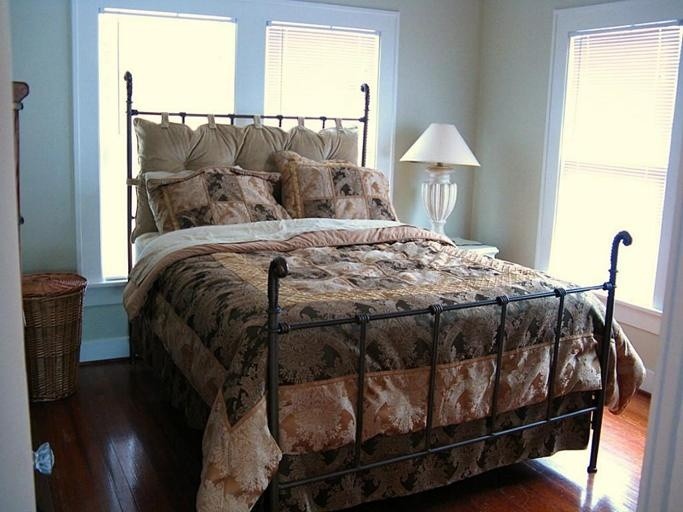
[142,150,401,235]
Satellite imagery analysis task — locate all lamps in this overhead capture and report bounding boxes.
[399,123,481,234]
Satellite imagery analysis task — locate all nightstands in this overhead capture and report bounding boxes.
[450,238,499,259]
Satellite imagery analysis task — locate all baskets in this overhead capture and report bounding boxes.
[22,274,87,403]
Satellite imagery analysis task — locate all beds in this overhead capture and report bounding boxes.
[123,72,633,512]
